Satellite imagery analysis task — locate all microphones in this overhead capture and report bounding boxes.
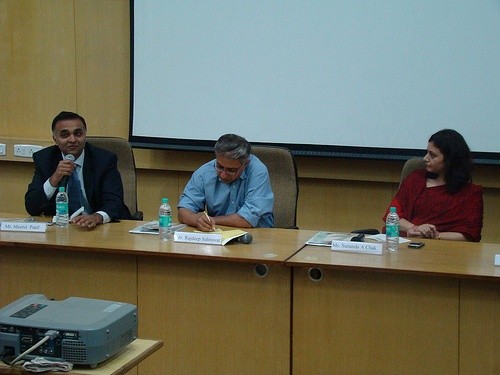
[351,233,366,243]
[231,233,253,243]
[59,154,75,188]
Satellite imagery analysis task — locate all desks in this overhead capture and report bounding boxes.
[0,308,166,375]
[0,212,500,375]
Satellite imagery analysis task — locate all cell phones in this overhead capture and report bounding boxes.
[408,242,424,248]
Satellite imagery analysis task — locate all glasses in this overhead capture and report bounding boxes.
[214,161,245,176]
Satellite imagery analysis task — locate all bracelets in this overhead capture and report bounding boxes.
[438,234,440,240]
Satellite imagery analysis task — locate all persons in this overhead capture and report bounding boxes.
[24,111,132,228]
[382,129,484,242]
[177,134,276,232]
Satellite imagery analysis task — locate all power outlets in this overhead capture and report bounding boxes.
[14,145,45,159]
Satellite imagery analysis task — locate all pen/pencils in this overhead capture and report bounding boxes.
[203,211,216,230]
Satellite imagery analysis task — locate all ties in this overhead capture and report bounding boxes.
[67,169,82,217]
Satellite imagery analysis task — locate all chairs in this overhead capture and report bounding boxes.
[249,146,299,230]
[84,135,145,221]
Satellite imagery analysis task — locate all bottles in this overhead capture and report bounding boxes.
[56,187,69,227]
[158,197,172,241]
[386,207,400,252]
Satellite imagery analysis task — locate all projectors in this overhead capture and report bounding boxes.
[0,294,139,368]
